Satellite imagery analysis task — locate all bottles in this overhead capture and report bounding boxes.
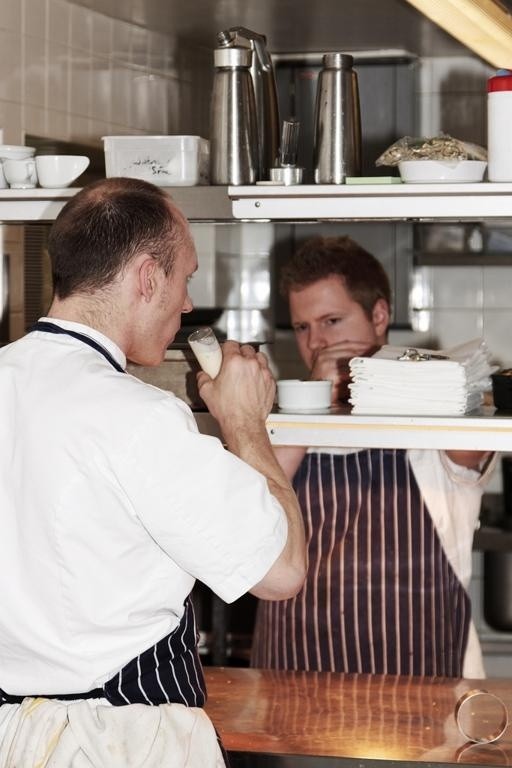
[312,50,364,186]
[207,26,281,187]
[487,75,512,183]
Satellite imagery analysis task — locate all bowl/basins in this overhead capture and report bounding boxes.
[271,378,333,410]
[398,159,489,184]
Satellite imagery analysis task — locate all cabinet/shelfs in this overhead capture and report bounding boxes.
[2,178,512,452]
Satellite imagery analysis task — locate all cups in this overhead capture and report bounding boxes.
[269,166,304,186]
[187,327,223,380]
[1,143,91,189]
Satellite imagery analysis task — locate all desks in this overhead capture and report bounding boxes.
[199,663,511,766]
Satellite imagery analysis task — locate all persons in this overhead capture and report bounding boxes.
[250,235,500,768]
[0,178,307,768]
